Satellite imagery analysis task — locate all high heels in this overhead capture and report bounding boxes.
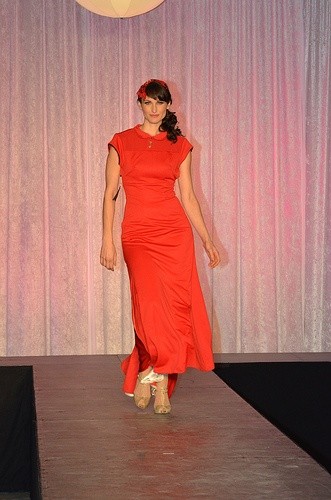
[153,386,172,414]
[133,372,151,409]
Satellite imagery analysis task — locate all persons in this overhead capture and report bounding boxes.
[100,78,220,414]
